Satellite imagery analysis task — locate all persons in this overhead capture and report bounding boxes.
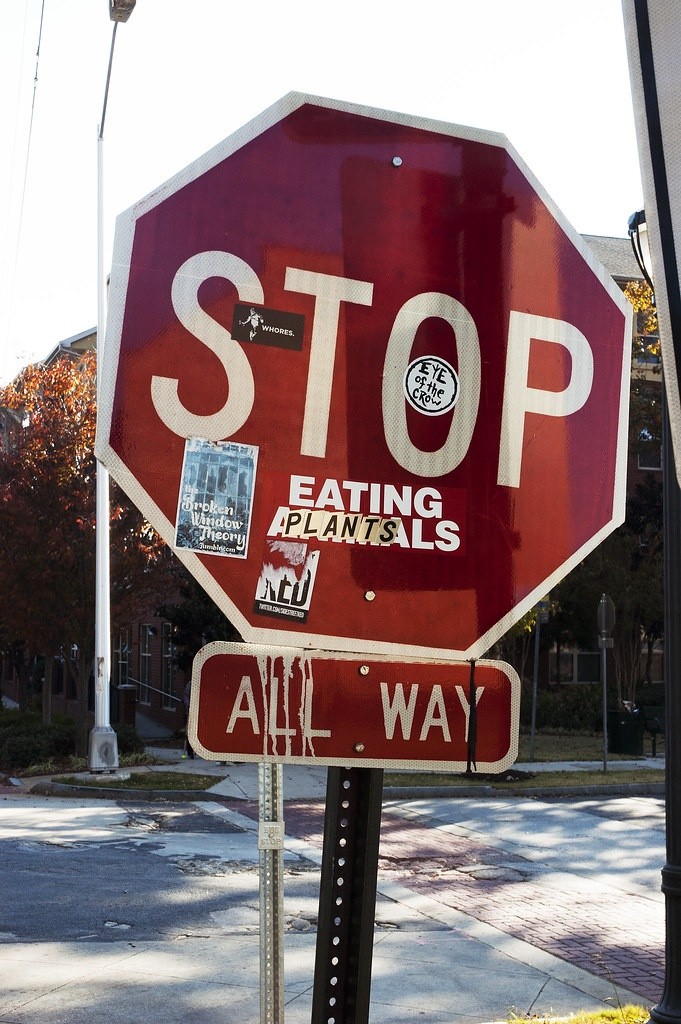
[184,679,246,766]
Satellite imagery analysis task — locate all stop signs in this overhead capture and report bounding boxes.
[91,89,635,657]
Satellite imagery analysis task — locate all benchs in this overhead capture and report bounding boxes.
[642,705,665,757]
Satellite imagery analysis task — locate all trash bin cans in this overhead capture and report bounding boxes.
[607,709,645,756]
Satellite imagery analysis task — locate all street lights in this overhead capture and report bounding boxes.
[86,0,139,780]
